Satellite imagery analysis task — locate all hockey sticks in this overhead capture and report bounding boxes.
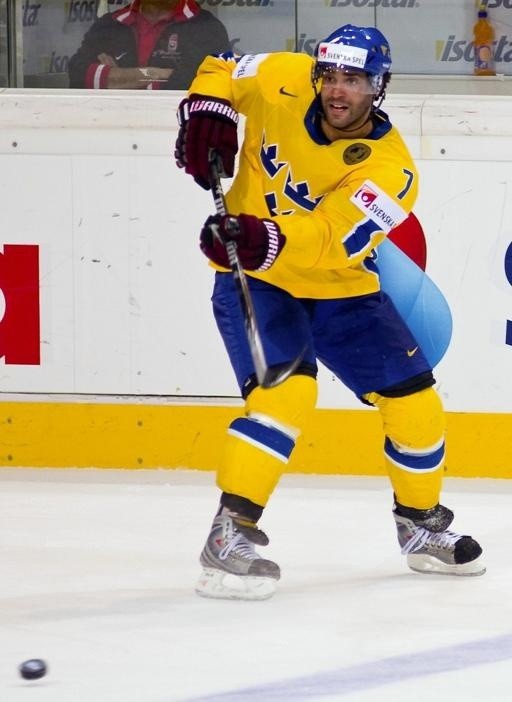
[208,161,310,387]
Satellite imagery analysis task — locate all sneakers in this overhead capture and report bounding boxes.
[199,515,281,580]
[392,505,483,564]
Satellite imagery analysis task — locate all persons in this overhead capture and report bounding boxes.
[67,0,232,89]
[172,27,483,580]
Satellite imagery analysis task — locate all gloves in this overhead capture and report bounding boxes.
[174,94,239,190]
[200,214,286,272]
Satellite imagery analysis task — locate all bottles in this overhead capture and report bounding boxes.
[471,8,497,78]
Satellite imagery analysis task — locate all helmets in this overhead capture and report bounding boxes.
[311,24,392,95]
